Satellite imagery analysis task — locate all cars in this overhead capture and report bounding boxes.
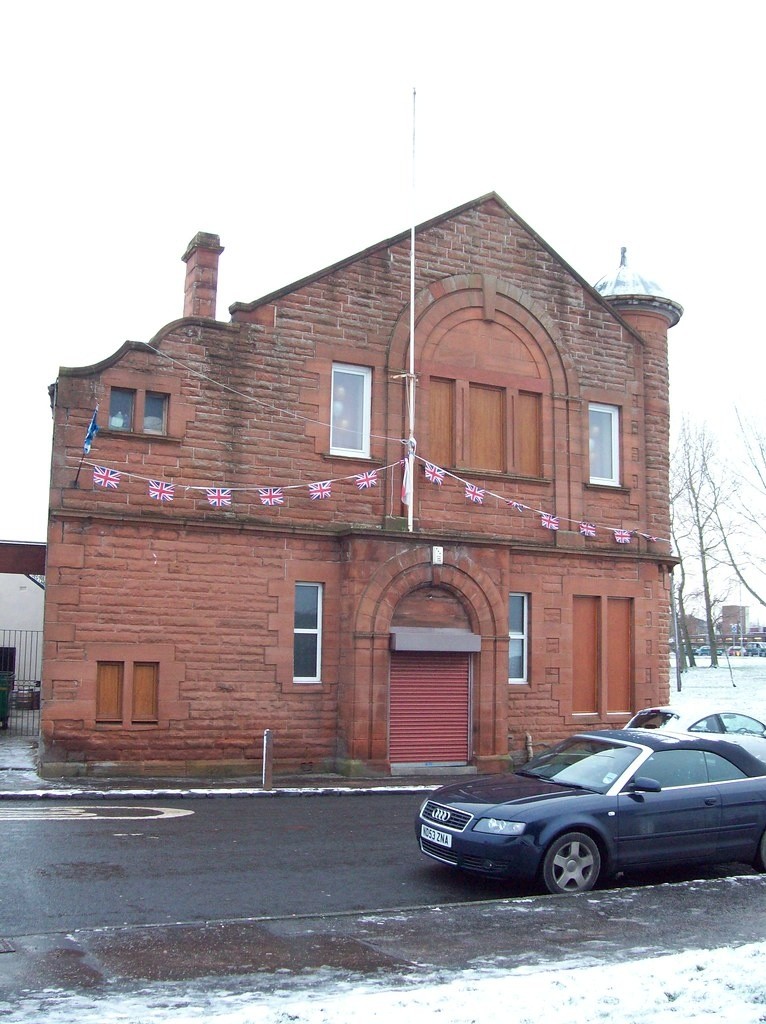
[728,646,746,657]
[414,729,766,892]
[611,708,766,745]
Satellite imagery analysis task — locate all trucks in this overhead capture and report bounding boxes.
[695,646,722,656]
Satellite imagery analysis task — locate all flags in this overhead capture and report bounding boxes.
[465,481,486,506]
[614,529,631,543]
[148,479,176,502]
[94,465,122,489]
[579,522,596,537]
[205,488,232,507]
[541,512,559,531]
[259,489,284,506]
[355,470,378,491]
[424,462,445,486]
[309,483,332,501]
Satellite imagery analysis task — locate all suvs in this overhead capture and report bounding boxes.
[745,642,766,658]
